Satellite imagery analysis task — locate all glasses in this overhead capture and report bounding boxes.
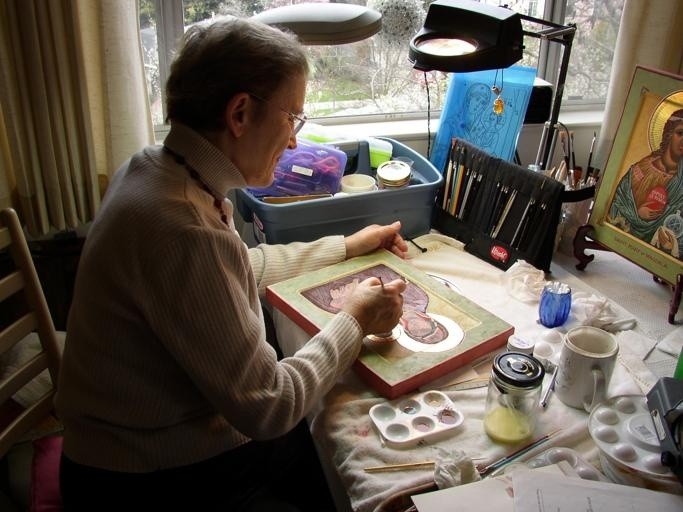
[249,92,308,140]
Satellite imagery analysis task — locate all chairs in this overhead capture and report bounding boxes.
[0,209,64,511]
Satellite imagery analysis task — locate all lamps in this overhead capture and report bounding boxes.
[247,2,382,44]
[407,0,576,177]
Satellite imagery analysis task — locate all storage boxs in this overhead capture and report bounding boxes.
[235,135,443,249]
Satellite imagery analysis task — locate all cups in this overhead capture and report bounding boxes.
[340,172,378,194]
[555,325,619,412]
[538,282,571,329]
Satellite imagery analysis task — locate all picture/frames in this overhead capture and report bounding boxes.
[585,65,683,285]
[265,250,517,404]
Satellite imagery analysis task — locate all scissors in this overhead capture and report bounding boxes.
[293,157,340,177]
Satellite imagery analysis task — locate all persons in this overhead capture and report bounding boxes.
[607,108,682,261]
[56,15,406,510]
[303,265,448,345]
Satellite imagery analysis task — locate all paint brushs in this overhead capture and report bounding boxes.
[559,131,572,181]
[585,131,596,185]
[442,138,486,222]
[492,431,564,469]
[510,179,547,248]
[570,131,576,189]
[365,458,488,472]
[483,180,518,239]
[378,276,385,285]
[478,426,562,474]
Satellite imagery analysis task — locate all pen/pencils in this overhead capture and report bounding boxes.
[540,366,558,407]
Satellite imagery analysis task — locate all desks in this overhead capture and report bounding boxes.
[253,223,682,512]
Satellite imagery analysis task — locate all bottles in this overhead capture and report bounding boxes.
[377,161,412,190]
[484,351,545,444]
[507,334,533,359]
